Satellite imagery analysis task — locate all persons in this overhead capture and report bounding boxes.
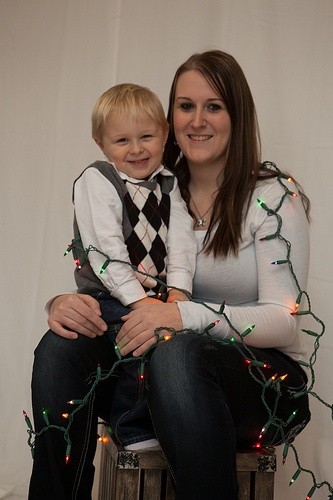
[26,47,318,499]
[70,80,200,454]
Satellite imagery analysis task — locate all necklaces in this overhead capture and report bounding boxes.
[187,189,214,227]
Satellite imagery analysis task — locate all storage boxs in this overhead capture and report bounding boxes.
[95,421,279,500]
